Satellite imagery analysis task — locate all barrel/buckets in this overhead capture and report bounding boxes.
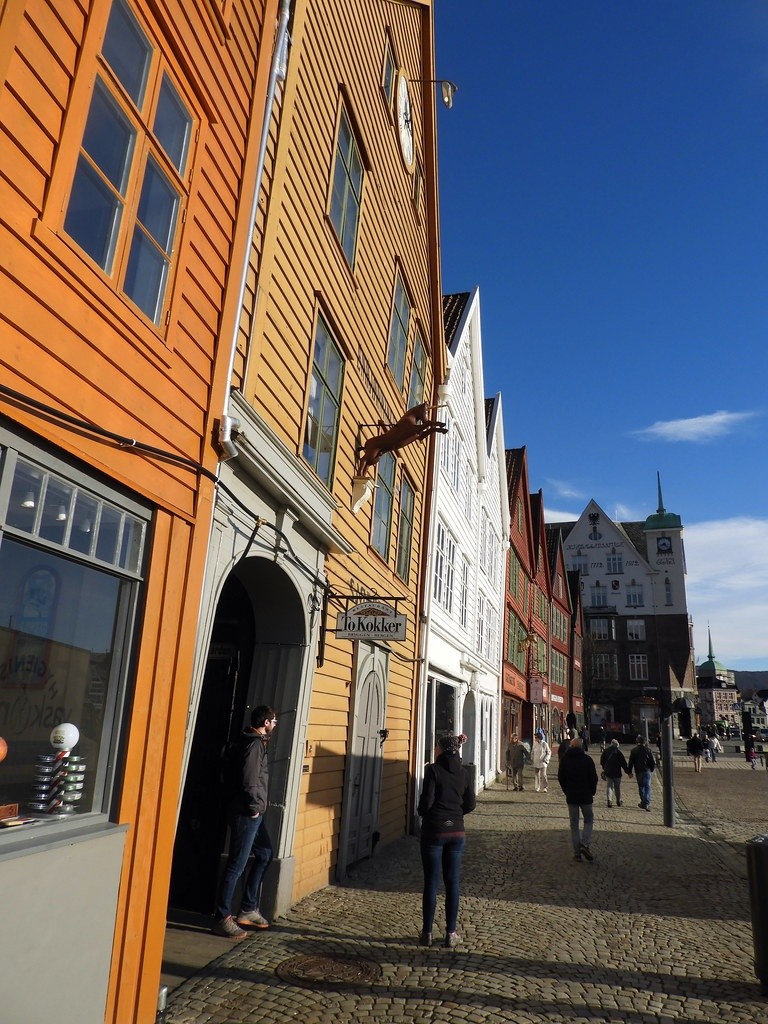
[745,835,768,991]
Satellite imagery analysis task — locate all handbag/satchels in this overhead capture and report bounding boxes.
[601,771,607,781]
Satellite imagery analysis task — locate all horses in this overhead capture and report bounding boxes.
[357,401,449,476]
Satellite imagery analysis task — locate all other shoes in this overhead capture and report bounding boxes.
[445,932,463,948]
[419,932,432,946]
[543,787,548,793]
[695,769,701,772]
[572,844,593,862]
[617,800,623,807]
[535,788,540,792]
[608,801,612,807]
[512,786,524,792]
[638,802,651,811]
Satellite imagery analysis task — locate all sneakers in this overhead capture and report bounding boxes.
[208,914,247,939]
[236,908,268,928]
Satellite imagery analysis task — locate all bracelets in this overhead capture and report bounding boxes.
[29,754,88,813]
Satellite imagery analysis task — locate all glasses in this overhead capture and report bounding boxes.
[535,737,538,738]
[271,720,277,722]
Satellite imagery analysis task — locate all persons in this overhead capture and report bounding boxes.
[505,734,530,792]
[685,733,722,773]
[529,732,552,793]
[628,735,656,812]
[600,738,633,808]
[558,724,590,761]
[557,738,598,862]
[211,704,279,940]
[748,748,757,770]
[415,734,476,949]
[599,725,606,753]
[656,732,663,761]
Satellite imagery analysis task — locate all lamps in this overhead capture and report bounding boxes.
[79,511,93,532]
[410,79,456,110]
[50,501,68,521]
[21,491,36,508]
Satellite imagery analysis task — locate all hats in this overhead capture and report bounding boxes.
[437,733,467,752]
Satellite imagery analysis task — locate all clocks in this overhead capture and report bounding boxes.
[396,67,416,173]
[657,538,670,550]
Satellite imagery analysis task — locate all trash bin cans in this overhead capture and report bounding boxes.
[745,833,768,989]
